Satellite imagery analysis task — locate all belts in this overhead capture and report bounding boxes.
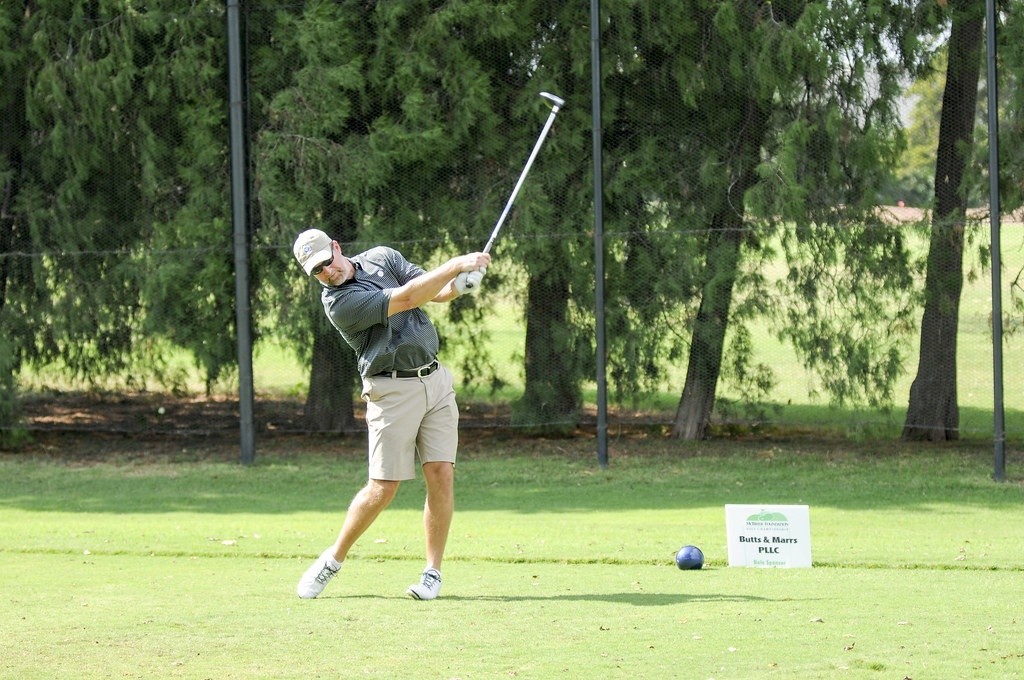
[373,357,440,378]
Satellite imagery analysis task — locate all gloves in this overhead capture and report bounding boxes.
[455,264,486,296]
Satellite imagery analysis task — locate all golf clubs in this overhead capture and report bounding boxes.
[466,91,567,290]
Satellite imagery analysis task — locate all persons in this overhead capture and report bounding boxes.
[293,228,491,600]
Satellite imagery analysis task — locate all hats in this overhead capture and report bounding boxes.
[292,228,333,277]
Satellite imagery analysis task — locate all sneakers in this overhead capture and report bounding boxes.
[297,546,344,599]
[408,569,441,599]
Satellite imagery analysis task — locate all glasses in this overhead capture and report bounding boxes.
[310,241,334,275]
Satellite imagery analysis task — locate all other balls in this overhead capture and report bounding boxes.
[676,545,704,569]
